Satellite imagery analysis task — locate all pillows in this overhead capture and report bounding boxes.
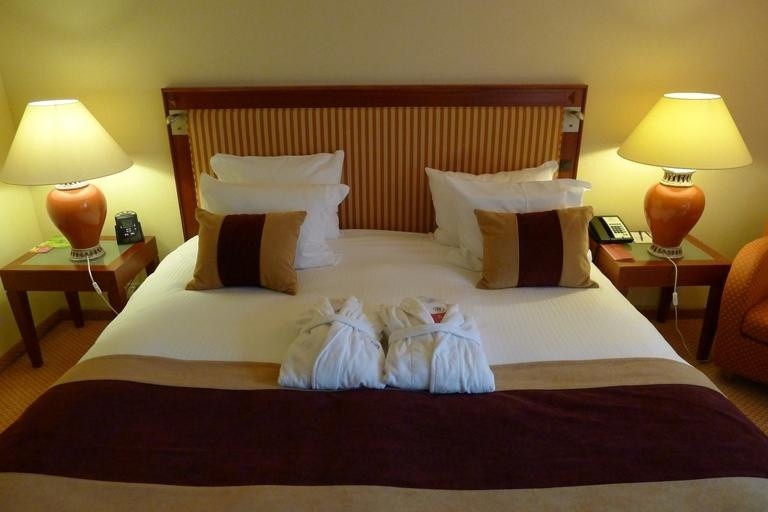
[424,160,600,290]
[184,149,351,297]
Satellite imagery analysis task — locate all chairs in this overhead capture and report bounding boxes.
[714,217,768,384]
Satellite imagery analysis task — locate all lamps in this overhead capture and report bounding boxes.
[0,100,135,262]
[617,93,754,258]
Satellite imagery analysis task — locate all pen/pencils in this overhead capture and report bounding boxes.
[639,231,643,241]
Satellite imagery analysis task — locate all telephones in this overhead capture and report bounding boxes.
[589,215,633,244]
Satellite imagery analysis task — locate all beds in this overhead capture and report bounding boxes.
[0,85,768,512]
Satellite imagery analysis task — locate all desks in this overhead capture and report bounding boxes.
[590,232,733,362]
[0,235,160,368]
